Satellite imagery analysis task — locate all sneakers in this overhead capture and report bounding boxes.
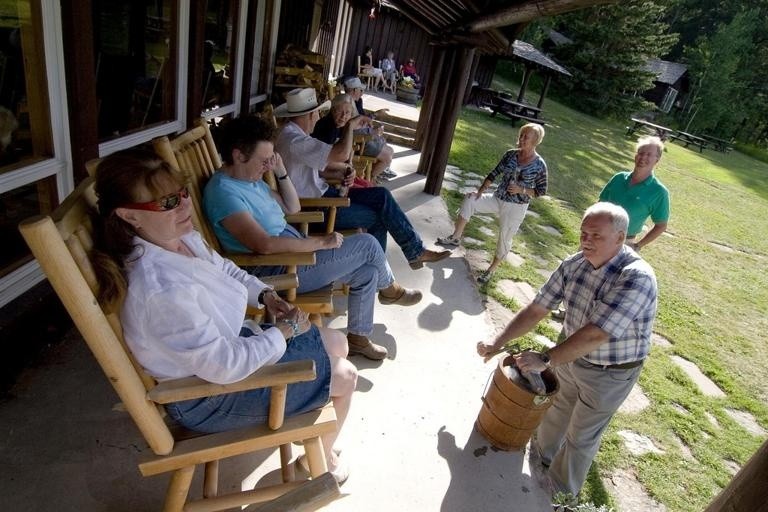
[437,235,460,246]
[295,454,349,483]
[377,168,396,178]
[376,176,385,185]
[477,270,496,283]
[551,308,565,319]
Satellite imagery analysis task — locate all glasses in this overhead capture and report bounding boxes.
[119,186,189,212]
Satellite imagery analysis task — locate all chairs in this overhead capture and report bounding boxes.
[18,158,340,512]
[358,56,405,95]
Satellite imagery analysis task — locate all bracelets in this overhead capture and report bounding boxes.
[523,186,526,194]
[634,244,641,250]
[258,290,269,304]
[278,175,288,181]
[281,319,301,337]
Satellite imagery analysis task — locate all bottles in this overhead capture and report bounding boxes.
[337,167,351,197]
[509,168,517,197]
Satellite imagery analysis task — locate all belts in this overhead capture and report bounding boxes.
[593,360,642,369]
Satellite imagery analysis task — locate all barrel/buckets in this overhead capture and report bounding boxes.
[477,347,561,450]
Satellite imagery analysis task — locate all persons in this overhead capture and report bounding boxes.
[437,123,547,283]
[272,86,453,269]
[310,45,420,187]
[89,146,359,483]
[201,114,422,362]
[478,201,657,511]
[598,135,669,254]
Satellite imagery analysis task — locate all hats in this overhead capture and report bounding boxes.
[273,87,332,118]
[345,77,366,89]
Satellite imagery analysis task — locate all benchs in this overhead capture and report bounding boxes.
[467,85,547,128]
[624,118,732,155]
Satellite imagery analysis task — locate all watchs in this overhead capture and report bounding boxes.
[541,353,551,369]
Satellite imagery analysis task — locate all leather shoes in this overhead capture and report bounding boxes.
[409,248,453,270]
[348,340,388,360]
[378,287,423,306]
